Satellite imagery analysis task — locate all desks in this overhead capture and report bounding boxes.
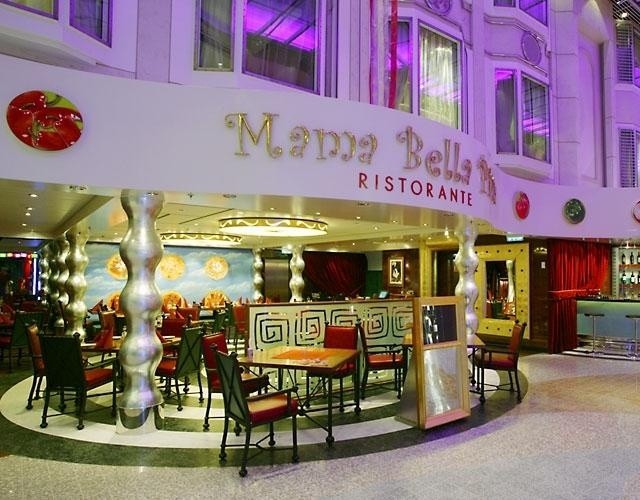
[235,346,358,444]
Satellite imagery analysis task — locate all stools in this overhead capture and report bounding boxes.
[625,315,640,358]
[584,313,604,353]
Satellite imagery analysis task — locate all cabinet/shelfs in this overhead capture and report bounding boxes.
[619,264,640,288]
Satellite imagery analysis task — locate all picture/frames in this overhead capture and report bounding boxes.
[387,256,404,288]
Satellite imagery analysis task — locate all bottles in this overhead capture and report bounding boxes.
[637,251,640,264]
[622,271,626,284]
[630,271,635,284]
[638,271,640,284]
[622,253,626,265]
[630,252,635,264]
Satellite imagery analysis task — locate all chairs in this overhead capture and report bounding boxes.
[305,322,358,414]
[37,335,116,430]
[24,324,87,412]
[1,301,249,364]
[210,345,297,477]
[468,319,527,406]
[153,327,203,410]
[360,323,405,399]
[202,332,254,436]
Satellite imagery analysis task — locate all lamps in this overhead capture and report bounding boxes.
[218,218,329,236]
[161,234,241,246]
